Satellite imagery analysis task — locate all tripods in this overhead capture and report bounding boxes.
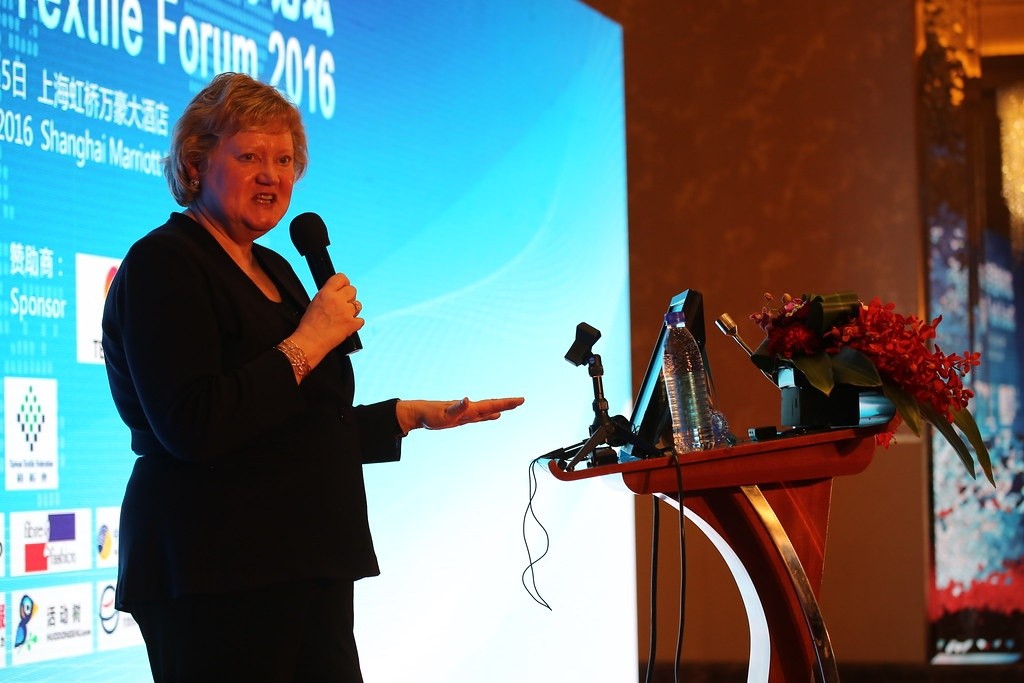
[558,322,666,472]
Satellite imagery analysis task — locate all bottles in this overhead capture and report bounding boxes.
[662,310,715,454]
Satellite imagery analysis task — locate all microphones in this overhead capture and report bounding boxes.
[290,212,363,355]
[713,313,782,388]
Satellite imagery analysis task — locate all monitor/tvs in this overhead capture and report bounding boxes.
[631,290,701,444]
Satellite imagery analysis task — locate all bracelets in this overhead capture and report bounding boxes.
[275,338,312,380]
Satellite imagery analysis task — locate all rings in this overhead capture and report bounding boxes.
[352,301,359,316]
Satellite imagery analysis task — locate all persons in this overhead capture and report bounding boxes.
[102,71,525,683]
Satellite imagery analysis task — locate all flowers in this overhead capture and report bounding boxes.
[750,291,996,490]
